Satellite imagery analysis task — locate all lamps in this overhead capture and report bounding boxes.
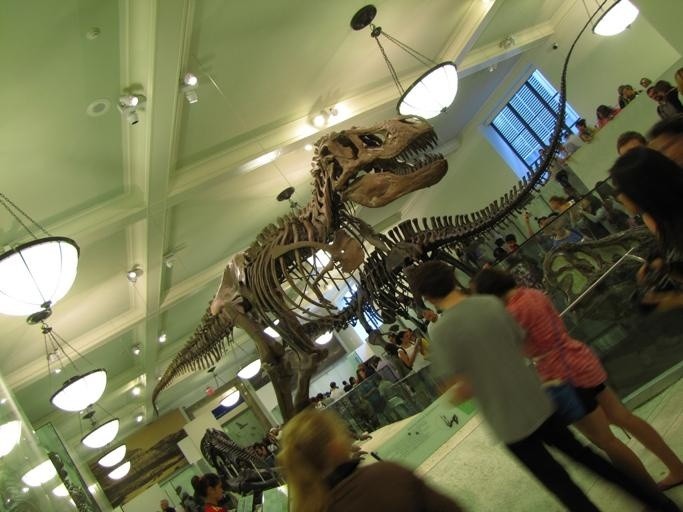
[208,309,333,410]
[276,186,332,268]
[38,322,130,480]
[577,0,639,39]
[348,4,459,123]
[1,194,80,325]
[178,73,198,104]
[162,248,176,269]
[114,87,140,126]
[3,391,70,497]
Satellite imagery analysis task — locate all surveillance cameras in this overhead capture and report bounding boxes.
[119,109,137,125]
[552,43,558,50]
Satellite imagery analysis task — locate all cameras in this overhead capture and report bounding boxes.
[567,199,575,206]
[525,210,532,218]
